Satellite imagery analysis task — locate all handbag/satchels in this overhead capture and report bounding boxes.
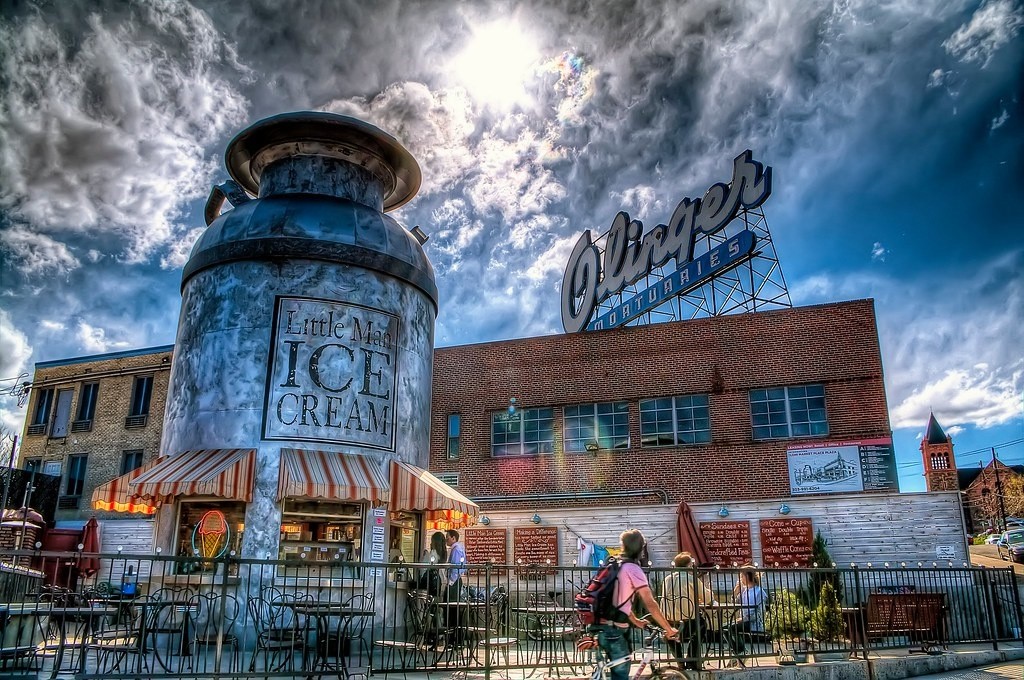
[420,569,442,597]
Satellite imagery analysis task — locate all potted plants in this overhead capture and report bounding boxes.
[763,586,811,664]
[810,580,851,663]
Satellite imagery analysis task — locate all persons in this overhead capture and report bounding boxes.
[419,530,466,651]
[588,529,680,680]
[720,565,767,668]
[661,552,715,673]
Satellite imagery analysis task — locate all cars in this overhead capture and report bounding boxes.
[967,534,974,544]
[985,533,1002,545]
[1006,517,1024,527]
[997,528,1024,563]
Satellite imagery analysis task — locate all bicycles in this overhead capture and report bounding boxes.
[523,591,587,641]
[540,621,693,680]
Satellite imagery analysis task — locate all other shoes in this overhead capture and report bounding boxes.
[726,648,751,668]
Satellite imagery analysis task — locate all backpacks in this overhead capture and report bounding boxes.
[575,556,640,626]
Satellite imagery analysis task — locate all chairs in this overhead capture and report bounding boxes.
[36,589,94,675]
[74,582,202,680]
[246,584,315,680]
[655,595,707,672]
[461,586,525,680]
[367,597,431,680]
[739,591,781,667]
[179,591,239,680]
[312,592,374,677]
[0,584,65,675]
[529,591,583,671]
[407,591,454,668]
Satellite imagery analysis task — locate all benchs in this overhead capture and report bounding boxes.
[861,592,949,653]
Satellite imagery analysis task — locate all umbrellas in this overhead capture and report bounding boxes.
[75,516,101,621]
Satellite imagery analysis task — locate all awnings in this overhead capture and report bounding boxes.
[126,448,258,504]
[276,447,392,503]
[91,454,168,515]
[388,458,479,530]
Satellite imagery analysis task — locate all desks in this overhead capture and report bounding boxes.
[512,606,591,678]
[293,608,377,680]
[427,602,499,667]
[30,608,118,680]
[269,601,350,672]
[699,603,758,668]
[99,599,199,675]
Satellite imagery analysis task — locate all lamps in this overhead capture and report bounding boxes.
[584,439,600,457]
[719,502,729,518]
[779,498,791,515]
[479,510,490,525]
[529,508,541,524]
